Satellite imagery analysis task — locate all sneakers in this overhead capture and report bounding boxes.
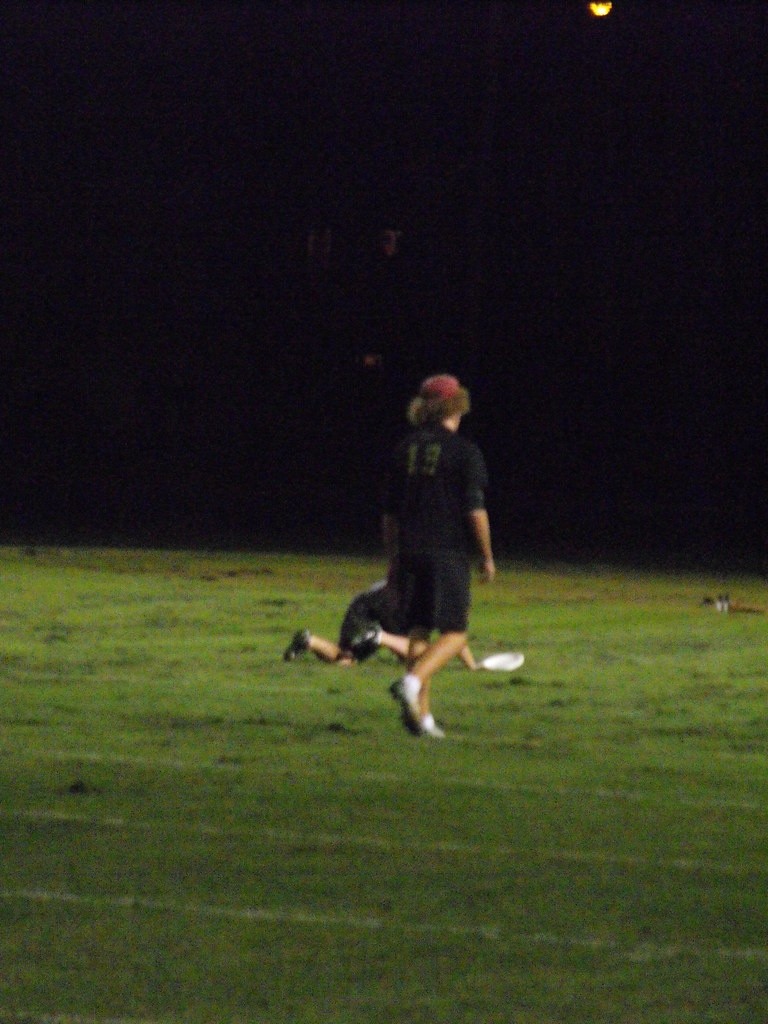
[390,673,426,738]
[402,711,447,740]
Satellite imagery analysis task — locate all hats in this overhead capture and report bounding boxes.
[406,373,474,426]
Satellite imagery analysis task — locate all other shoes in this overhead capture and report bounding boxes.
[283,628,311,662]
[353,622,384,662]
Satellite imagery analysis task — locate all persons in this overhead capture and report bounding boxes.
[282,575,482,671]
[389,375,496,737]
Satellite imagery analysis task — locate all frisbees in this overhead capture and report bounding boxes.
[482,650,526,673]
